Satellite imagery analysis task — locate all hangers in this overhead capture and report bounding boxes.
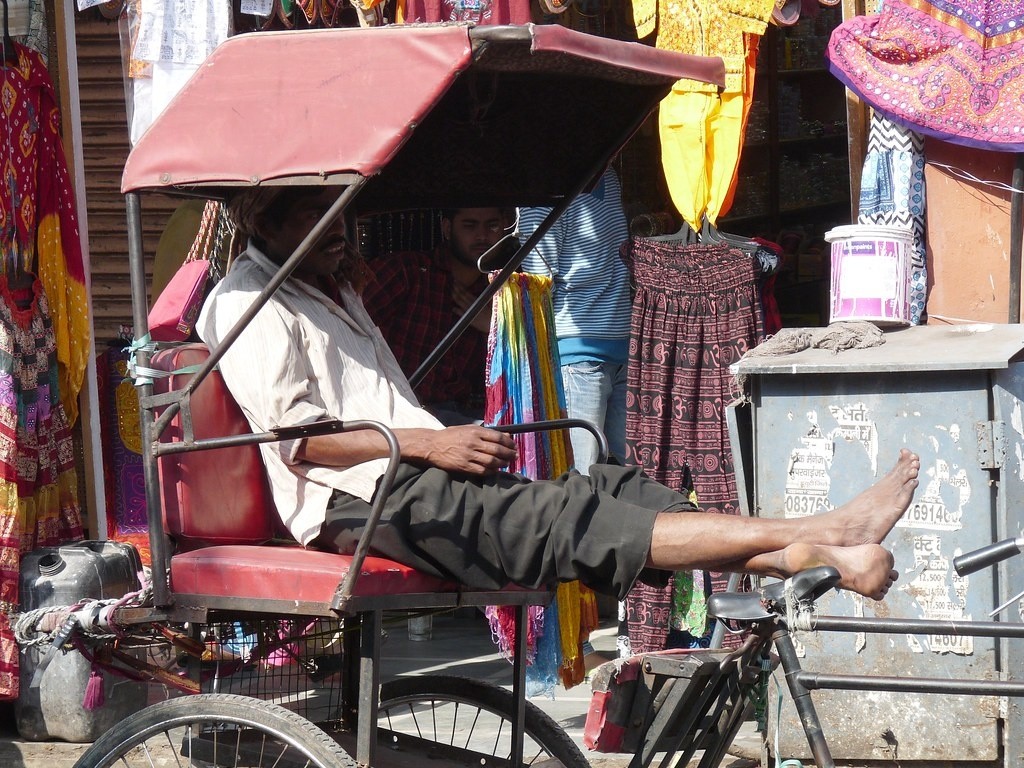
[640,210,764,257]
[477,206,553,277]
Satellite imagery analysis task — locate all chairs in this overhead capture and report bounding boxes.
[147,342,441,603]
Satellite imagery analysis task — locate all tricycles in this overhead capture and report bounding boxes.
[11,20,1023,768]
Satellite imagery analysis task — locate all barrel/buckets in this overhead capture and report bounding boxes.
[826,225,914,328]
[17,533,145,742]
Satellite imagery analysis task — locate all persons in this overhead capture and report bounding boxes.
[195,186,921,601]
[350,163,632,479]
